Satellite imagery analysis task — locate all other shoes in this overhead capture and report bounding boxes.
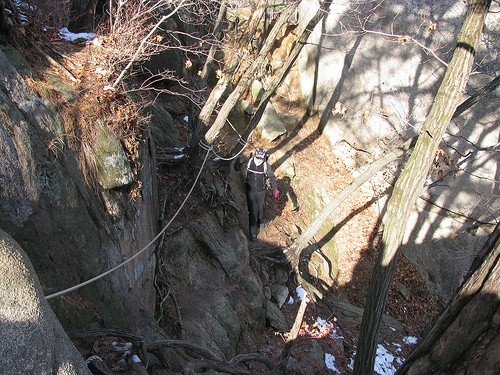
[260,220,267,227]
[251,234,257,241]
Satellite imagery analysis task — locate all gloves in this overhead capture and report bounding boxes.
[273,188,280,198]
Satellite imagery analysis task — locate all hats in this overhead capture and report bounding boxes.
[255,148,266,158]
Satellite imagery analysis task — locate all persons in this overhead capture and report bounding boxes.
[232,148,280,242]
